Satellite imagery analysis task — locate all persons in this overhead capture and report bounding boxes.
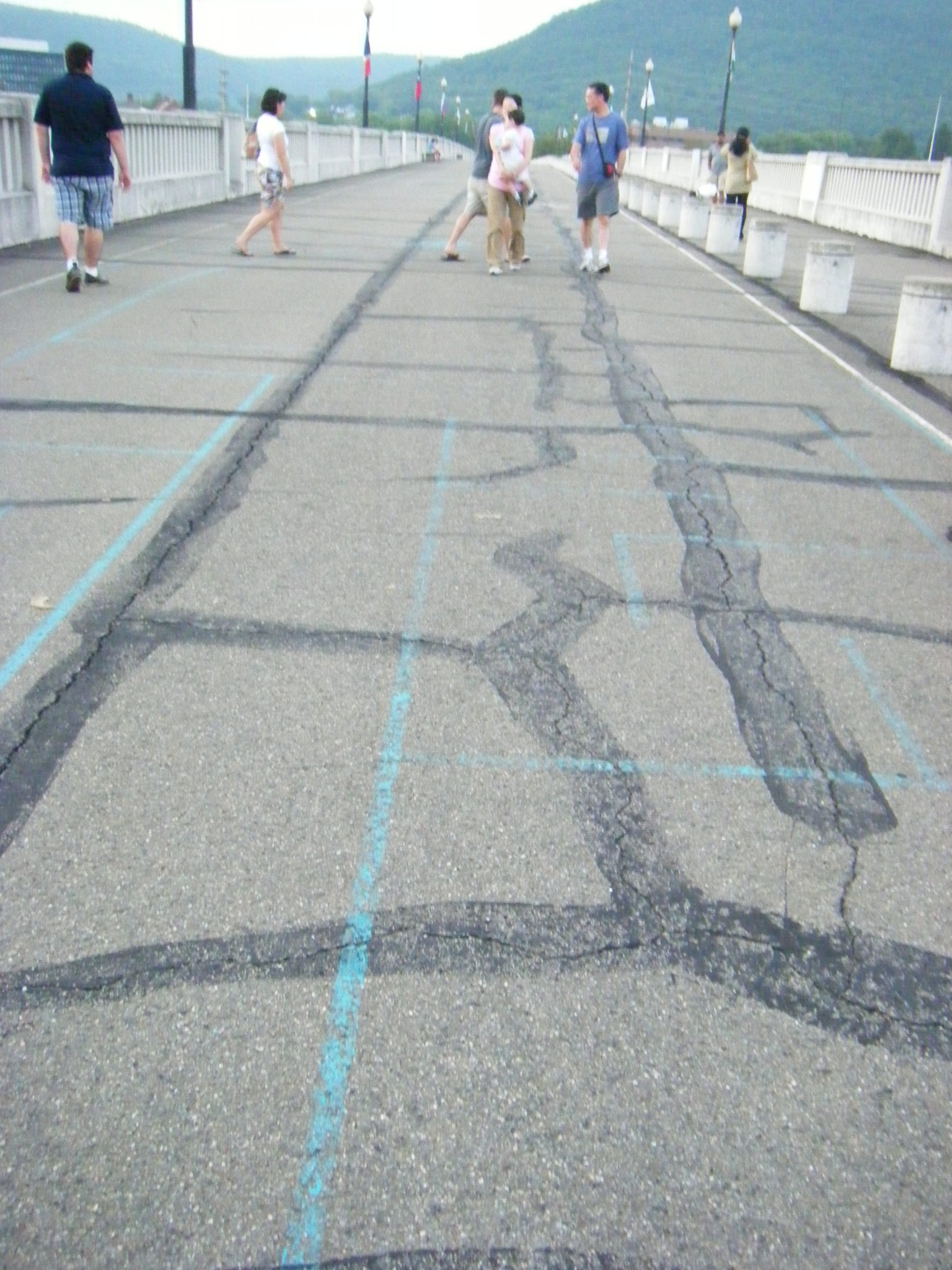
[441,92,530,262]
[236,88,297,256]
[428,138,440,165]
[707,130,731,205]
[487,93,534,275]
[570,82,628,272]
[499,108,537,205]
[718,127,757,240]
[34,41,131,292]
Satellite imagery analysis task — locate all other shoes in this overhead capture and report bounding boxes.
[526,191,537,206]
[517,191,523,206]
[580,258,594,272]
[440,246,465,261]
[488,265,503,275]
[505,254,530,263]
[594,259,610,273]
[509,261,520,272]
[85,271,109,286]
[66,262,82,293]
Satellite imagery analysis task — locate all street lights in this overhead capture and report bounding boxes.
[414,52,425,133]
[439,76,448,135]
[572,113,579,138]
[717,6,743,134]
[607,84,615,110]
[640,57,655,147]
[359,1,375,128]
[454,96,462,144]
[464,107,470,145]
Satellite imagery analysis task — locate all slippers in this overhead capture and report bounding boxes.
[274,246,297,256]
[230,246,254,257]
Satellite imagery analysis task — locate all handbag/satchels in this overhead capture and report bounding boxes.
[745,163,758,182]
[602,161,614,176]
[242,122,260,160]
